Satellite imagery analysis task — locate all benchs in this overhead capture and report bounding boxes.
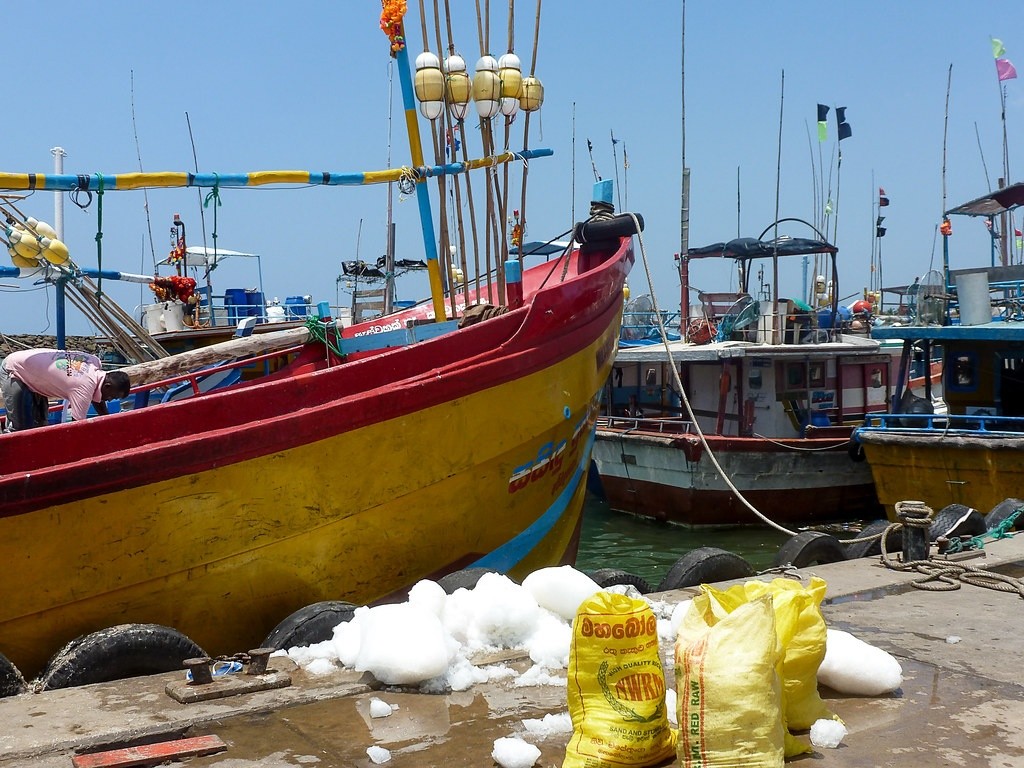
[697,293,760,341]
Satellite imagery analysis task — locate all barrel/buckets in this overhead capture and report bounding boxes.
[224,288,270,326]
[800,411,831,439]
[0,394,122,425]
[142,299,185,334]
[284,296,307,321]
[816,306,851,329]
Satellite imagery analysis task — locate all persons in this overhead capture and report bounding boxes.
[0,347,131,431]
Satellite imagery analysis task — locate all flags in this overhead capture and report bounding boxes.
[818,121,827,140]
[877,217,886,237]
[836,107,847,123]
[817,104,830,121]
[1015,229,1024,248]
[880,188,889,206]
[838,123,852,141]
[992,39,1018,81]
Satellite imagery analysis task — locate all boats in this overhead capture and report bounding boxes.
[0,0,646,697]
[539,0,1024,530]
